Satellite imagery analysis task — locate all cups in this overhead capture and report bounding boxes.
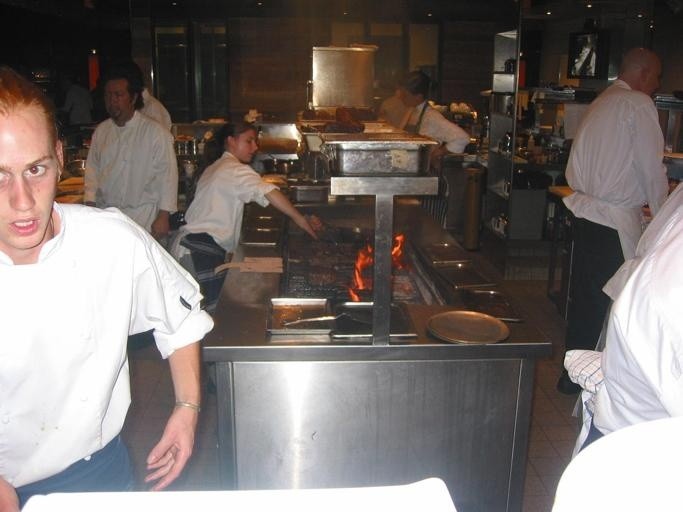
[184,164,198,175]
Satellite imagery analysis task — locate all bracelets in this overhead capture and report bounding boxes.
[173,401,202,416]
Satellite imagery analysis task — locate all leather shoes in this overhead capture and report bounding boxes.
[557,376,581,395]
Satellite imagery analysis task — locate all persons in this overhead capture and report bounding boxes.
[550,180,682,512]
[556,45,669,395]
[394,69,470,230]
[0,62,216,512]
[103,56,173,135]
[51,73,95,127]
[377,63,410,127]
[170,115,319,308]
[81,68,180,350]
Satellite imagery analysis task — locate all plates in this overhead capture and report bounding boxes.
[425,310,510,346]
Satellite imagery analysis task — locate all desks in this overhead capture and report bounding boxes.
[545,182,580,322]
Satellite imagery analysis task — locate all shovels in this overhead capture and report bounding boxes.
[283,307,373,327]
